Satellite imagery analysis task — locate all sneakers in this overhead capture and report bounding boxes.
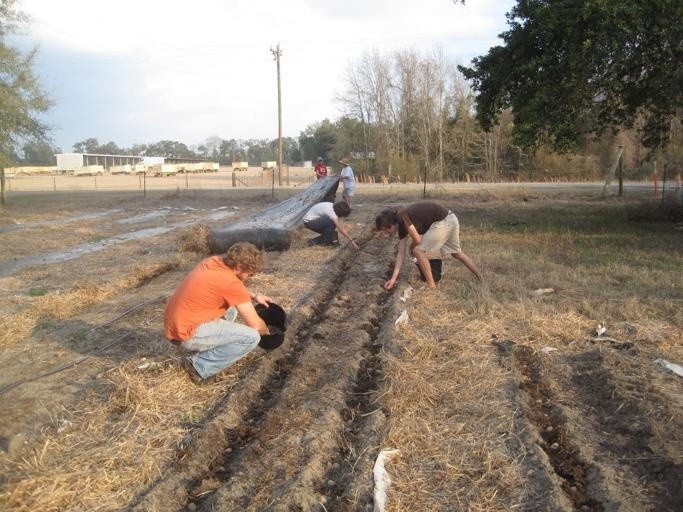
[180,356,203,387]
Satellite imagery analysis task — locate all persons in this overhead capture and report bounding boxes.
[374,202,484,292]
[314,156,327,182]
[160,241,273,388]
[301,201,359,249]
[337,159,355,209]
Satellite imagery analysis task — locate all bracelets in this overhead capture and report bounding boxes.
[252,294,257,300]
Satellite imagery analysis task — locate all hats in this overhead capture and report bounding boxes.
[316,156,323,162]
[338,158,352,166]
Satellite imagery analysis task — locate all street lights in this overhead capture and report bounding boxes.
[269,40,286,186]
